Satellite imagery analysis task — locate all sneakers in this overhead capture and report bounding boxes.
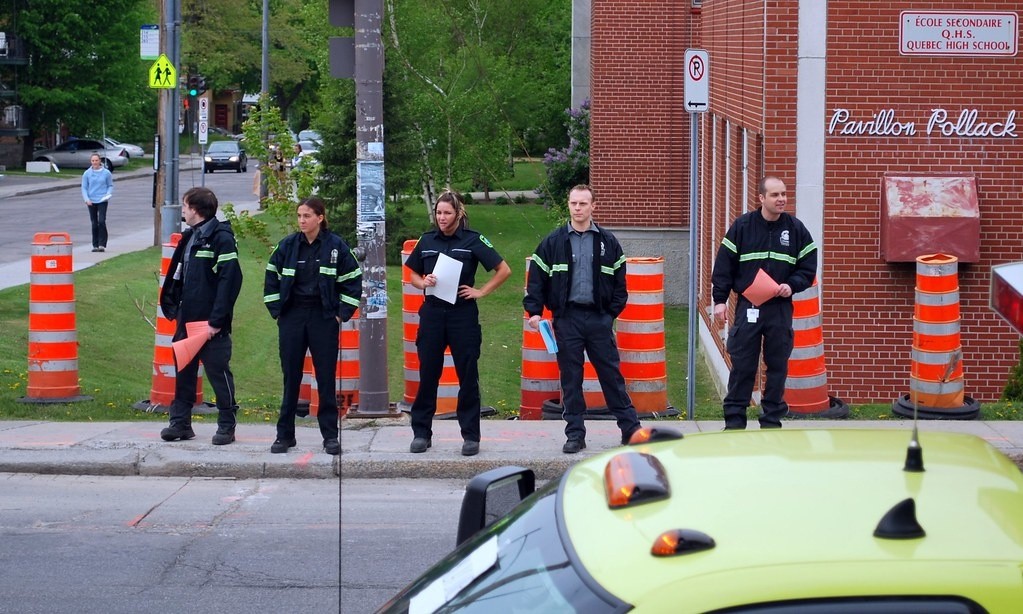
[272,437,296,454]
[322,438,341,454]
[211,425,236,444]
[161,425,195,441]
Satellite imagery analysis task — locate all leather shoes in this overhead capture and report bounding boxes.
[409,435,432,453]
[563,438,587,453]
[460,439,480,456]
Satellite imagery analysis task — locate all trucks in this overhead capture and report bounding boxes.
[373,262,1023,614]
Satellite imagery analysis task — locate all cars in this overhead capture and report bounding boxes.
[179,122,333,171]
[33,137,128,173]
[204,141,248,173]
[105,137,144,159]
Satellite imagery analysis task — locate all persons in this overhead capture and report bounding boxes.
[711,175,818,434]
[80,154,114,253]
[263,196,363,456]
[271,142,288,197]
[522,183,653,455]
[252,140,279,211]
[292,144,307,188]
[161,184,244,446]
[405,189,512,456]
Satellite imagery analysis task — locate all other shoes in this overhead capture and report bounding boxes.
[91,245,105,252]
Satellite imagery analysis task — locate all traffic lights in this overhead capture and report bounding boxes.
[184,95,198,111]
[198,76,207,96]
[187,73,198,96]
